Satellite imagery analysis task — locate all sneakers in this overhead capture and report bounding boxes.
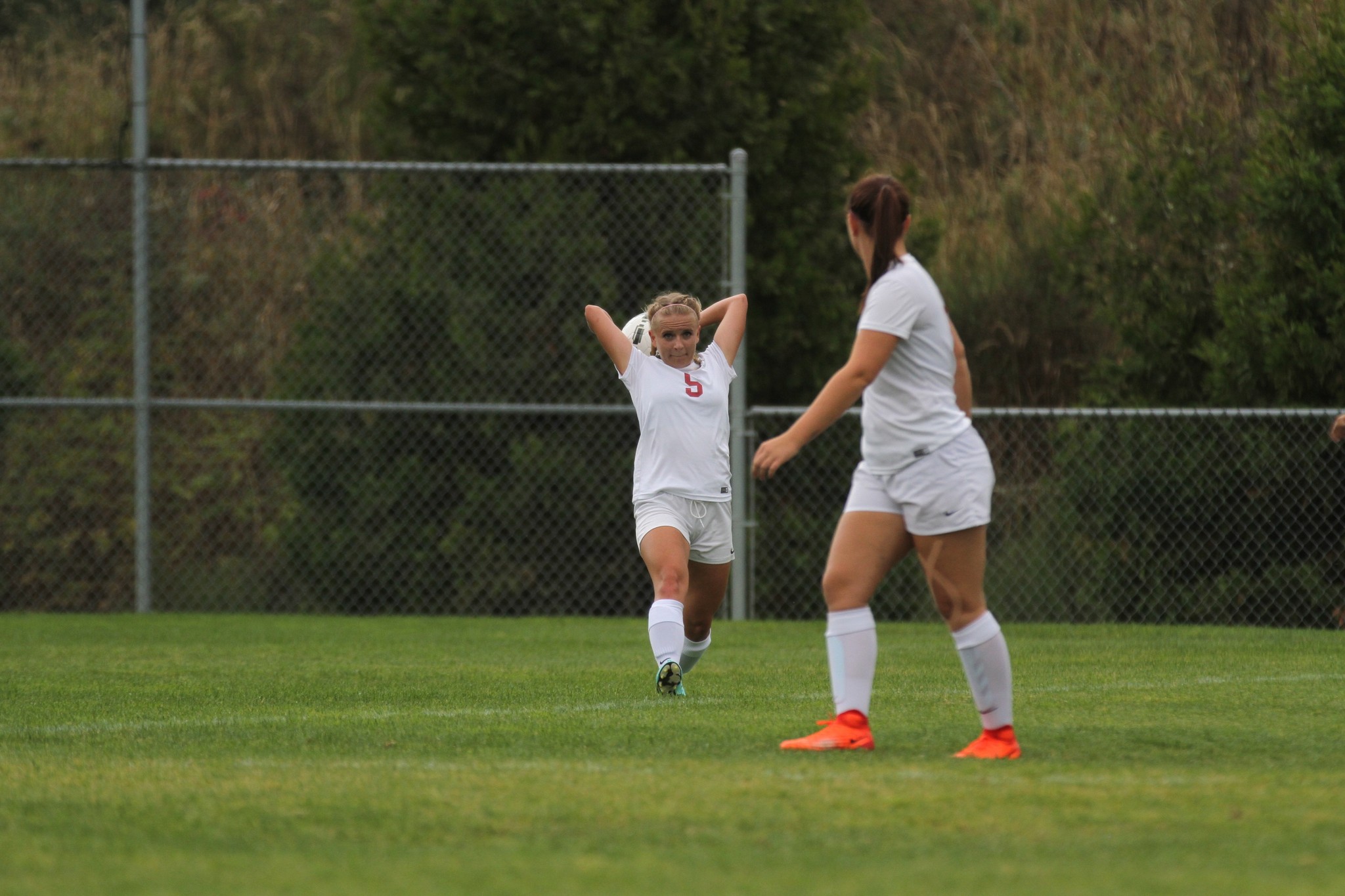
[955,724,1020,758]
[657,661,689,695]
[779,709,876,753]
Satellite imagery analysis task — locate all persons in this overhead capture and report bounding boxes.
[584,293,747,692]
[751,175,1020,759]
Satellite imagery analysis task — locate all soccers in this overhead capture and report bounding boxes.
[620,312,661,359]
